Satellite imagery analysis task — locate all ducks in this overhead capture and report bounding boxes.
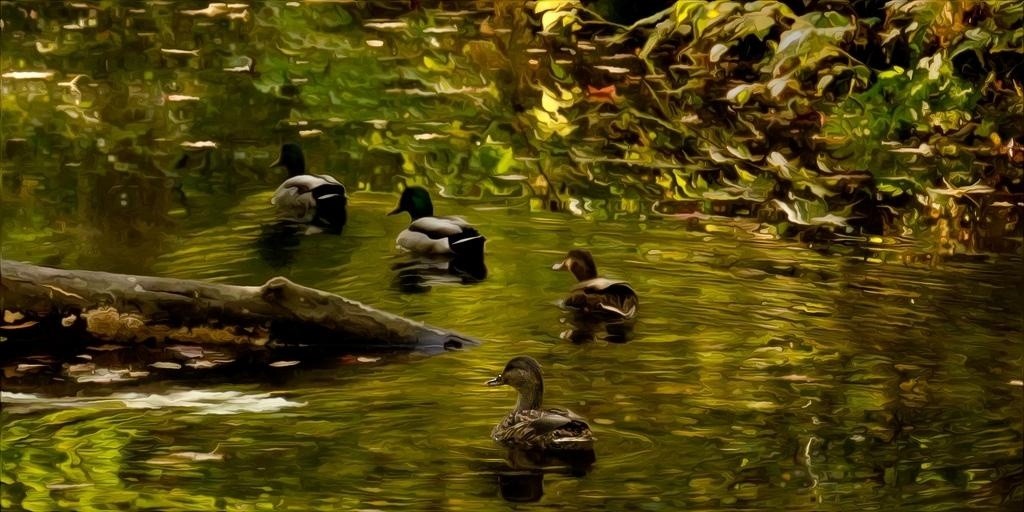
[387,186,485,253]
[267,142,347,207]
[484,354,598,450]
[551,249,637,315]
